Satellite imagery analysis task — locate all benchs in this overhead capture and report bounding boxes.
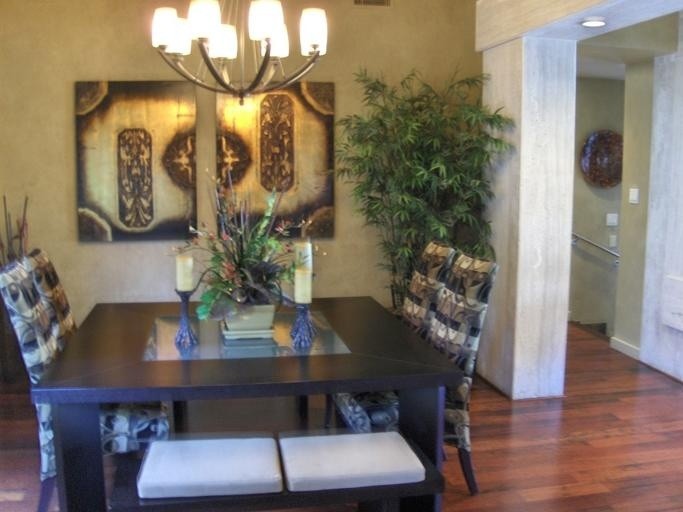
[110,430,447,511]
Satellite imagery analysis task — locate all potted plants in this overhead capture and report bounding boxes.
[189,163,314,343]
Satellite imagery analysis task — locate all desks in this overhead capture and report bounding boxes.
[33,296,464,512]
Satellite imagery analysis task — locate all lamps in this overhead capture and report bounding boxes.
[150,0,327,105]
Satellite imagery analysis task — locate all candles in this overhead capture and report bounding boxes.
[175,254,193,293]
[293,240,311,306]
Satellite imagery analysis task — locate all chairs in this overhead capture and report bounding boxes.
[25,248,166,409]
[324,241,455,430]
[0,261,170,511]
[338,254,501,495]
